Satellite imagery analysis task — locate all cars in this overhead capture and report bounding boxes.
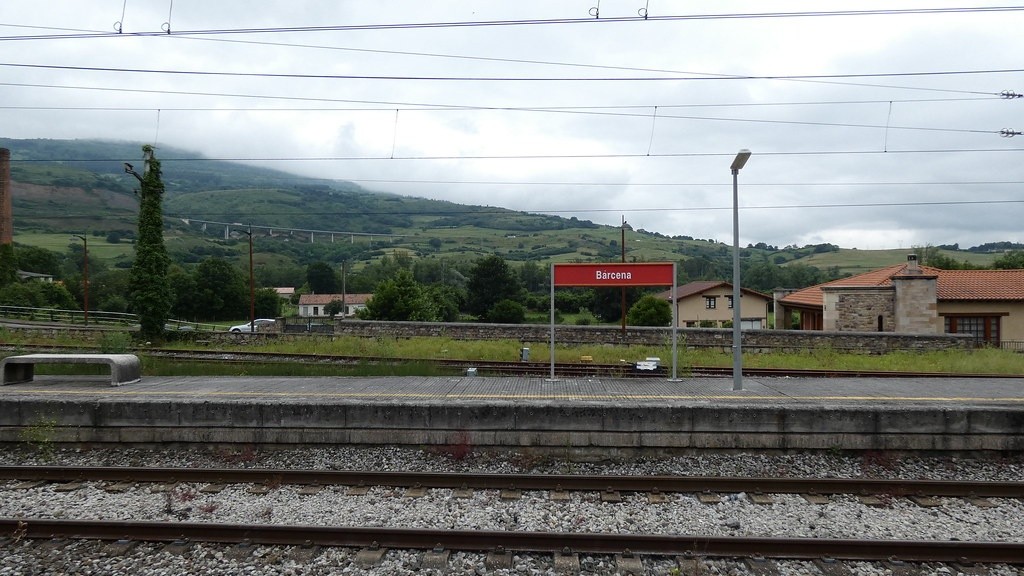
[228,318,275,334]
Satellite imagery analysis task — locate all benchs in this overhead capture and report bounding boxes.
[0,352,141,387]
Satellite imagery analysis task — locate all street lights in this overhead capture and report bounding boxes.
[730,148,752,391]
[72,232,88,327]
[233,222,254,332]
